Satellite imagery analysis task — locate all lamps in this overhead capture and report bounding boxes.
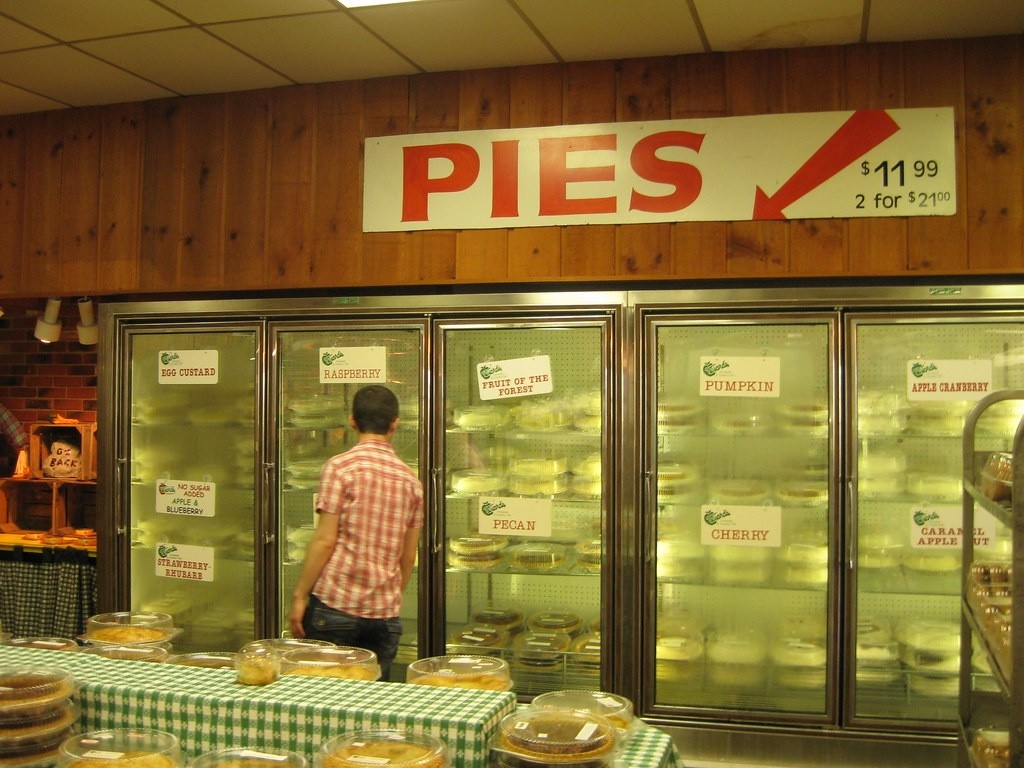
[34,298,64,344]
[75,297,99,345]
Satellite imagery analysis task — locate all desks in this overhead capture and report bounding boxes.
[0,643,517,768]
[512,702,686,768]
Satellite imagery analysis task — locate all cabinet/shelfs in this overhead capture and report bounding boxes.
[956,392,1024,767]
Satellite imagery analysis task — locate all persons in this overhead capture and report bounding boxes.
[288,386,423,682]
